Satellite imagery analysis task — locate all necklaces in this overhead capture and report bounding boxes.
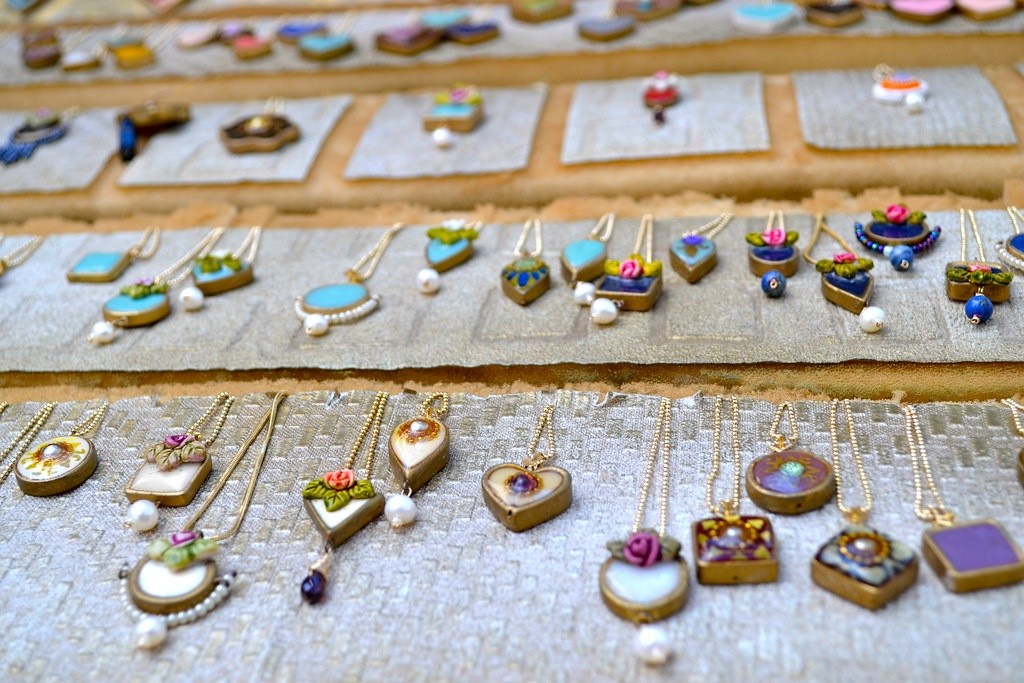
[746,400,836,514]
[0,233,45,274]
[68,228,160,282]
[0,1,1023,164]
[301,390,389,602]
[481,404,572,532]
[418,219,483,293]
[294,223,402,335]
[692,395,779,582]
[590,213,663,325]
[669,213,732,283]
[946,208,1013,324]
[904,404,1024,592]
[14,401,110,496]
[1001,397,1024,486]
[87,228,222,342]
[181,226,261,309]
[119,390,289,647]
[501,219,550,303]
[996,206,1024,271]
[0,401,57,484]
[125,392,234,531]
[384,392,449,529]
[746,210,799,296]
[812,398,919,608]
[561,214,614,304]
[803,211,885,333]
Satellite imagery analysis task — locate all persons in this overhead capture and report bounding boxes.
[599,396,689,663]
